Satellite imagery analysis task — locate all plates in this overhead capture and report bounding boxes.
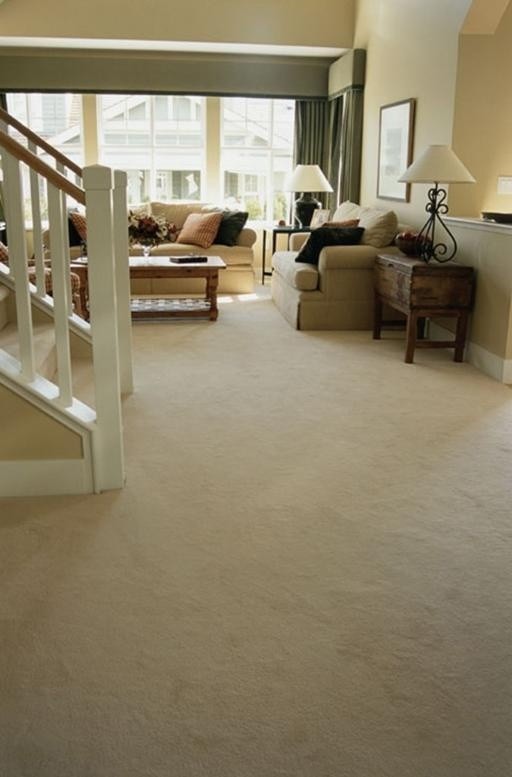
[481,211,512,223]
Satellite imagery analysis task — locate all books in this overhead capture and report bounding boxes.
[169,256,207,263]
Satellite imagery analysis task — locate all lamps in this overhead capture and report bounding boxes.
[286,164,334,226]
[396,144,476,264]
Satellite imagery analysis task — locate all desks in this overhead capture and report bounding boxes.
[373,253,475,364]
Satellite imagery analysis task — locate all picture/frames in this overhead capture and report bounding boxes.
[376,98,416,204]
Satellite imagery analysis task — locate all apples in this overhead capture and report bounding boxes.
[395,231,424,241]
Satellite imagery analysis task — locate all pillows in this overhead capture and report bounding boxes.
[68,203,249,250]
[294,200,398,262]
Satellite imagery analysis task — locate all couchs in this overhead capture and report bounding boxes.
[43,200,257,294]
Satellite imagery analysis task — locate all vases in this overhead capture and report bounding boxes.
[143,244,152,260]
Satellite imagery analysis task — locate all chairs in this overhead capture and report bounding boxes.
[0,241,89,320]
[270,200,417,331]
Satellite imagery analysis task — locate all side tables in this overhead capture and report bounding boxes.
[261,226,311,285]
